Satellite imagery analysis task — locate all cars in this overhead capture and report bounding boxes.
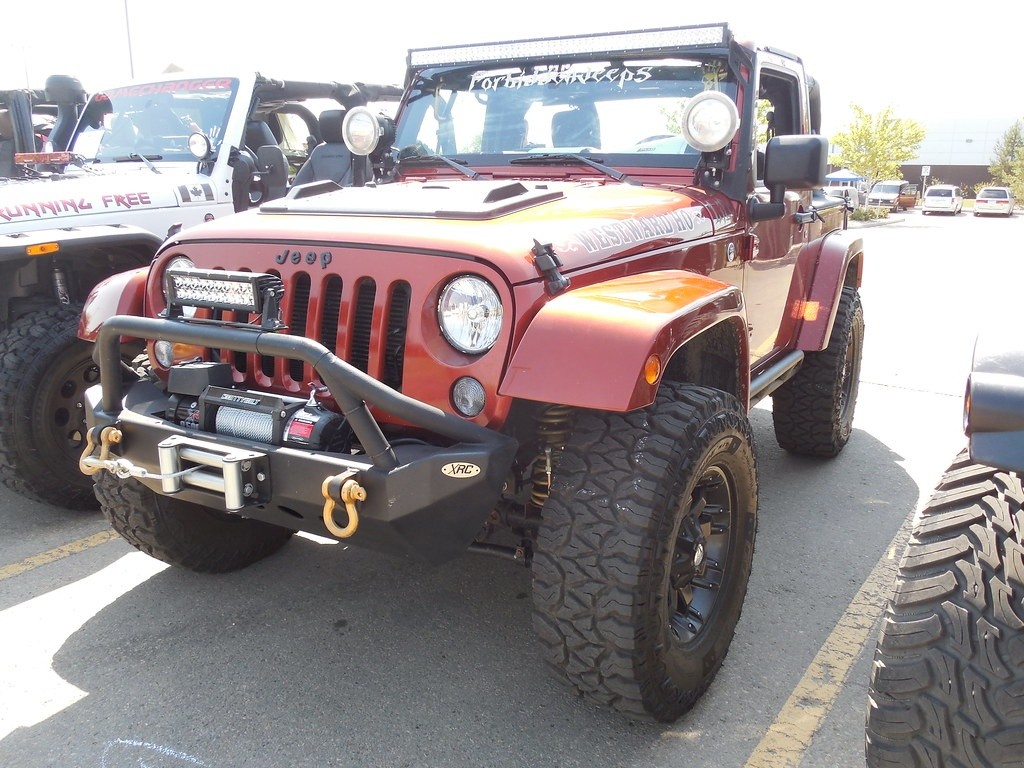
[921,184,963,216]
[973,187,1016,217]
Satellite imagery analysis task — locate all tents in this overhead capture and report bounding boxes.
[825,168,862,188]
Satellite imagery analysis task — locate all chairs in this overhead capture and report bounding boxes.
[104,116,136,156]
[0,109,43,178]
[287,109,374,192]
[246,120,278,156]
[551,110,602,150]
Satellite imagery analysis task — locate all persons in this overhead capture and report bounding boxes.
[468,297,490,348]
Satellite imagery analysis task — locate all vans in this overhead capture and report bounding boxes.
[822,186,860,213]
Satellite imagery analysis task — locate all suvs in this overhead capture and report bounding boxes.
[76,20,863,722]
[0,66,460,513]
[867,179,919,214]
